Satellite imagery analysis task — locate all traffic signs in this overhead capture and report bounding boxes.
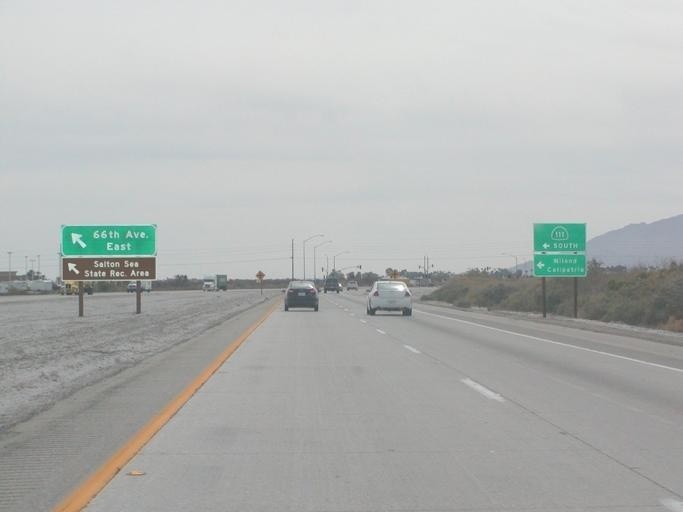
[59,223,157,282]
[532,221,588,279]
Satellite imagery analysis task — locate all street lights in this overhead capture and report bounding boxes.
[313,240,350,281]
[302,233,325,281]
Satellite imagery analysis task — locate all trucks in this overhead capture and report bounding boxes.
[126,281,152,293]
[215,274,227,291]
[202,278,216,291]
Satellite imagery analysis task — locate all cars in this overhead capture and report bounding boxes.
[280,280,319,312]
[366,280,413,316]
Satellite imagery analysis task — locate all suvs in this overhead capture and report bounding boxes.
[346,281,358,291]
[323,280,339,293]
